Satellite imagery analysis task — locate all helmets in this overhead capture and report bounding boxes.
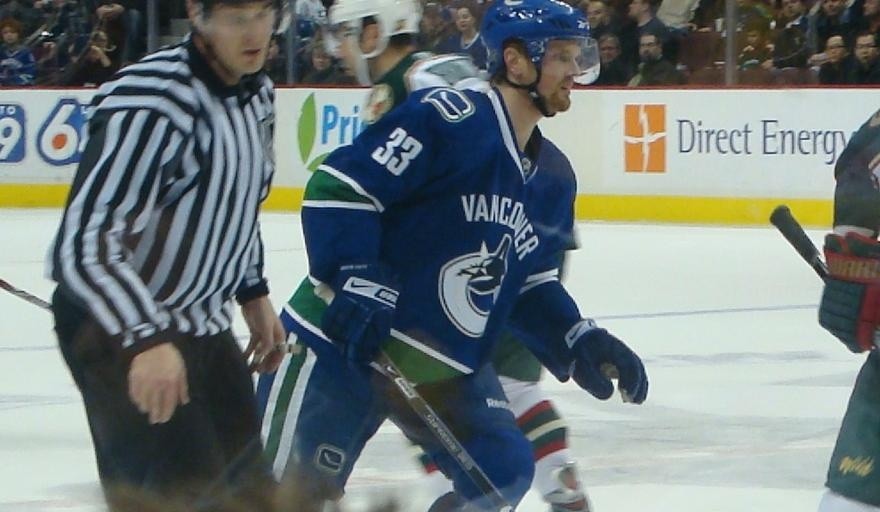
[327,2,422,44]
[479,2,592,75]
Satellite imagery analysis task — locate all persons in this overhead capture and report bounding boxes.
[253,1,651,512]
[51,1,288,512]
[817,104,880,512]
[2,0,880,89]
[327,1,594,512]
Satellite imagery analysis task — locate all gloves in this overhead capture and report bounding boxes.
[817,231,876,352]
[564,316,649,405]
[322,279,400,375]
